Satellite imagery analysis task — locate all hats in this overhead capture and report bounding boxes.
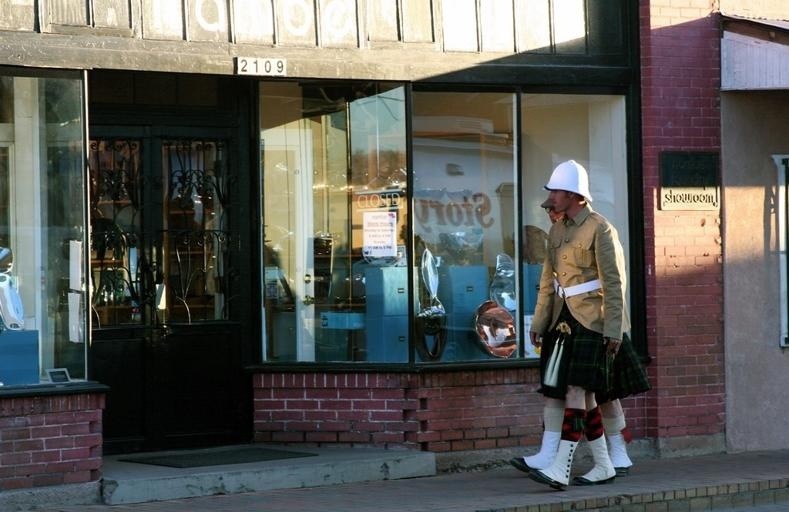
[540,160,593,208]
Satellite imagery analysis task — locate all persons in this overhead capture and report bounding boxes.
[508,193,638,474]
[523,157,630,491]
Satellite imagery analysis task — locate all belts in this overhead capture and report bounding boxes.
[553,278,602,299]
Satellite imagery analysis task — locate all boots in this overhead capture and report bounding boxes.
[513,432,579,491]
[572,430,633,485]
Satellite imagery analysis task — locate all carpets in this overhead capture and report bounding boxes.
[117,447,319,468]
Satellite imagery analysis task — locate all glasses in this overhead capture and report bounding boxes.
[545,208,555,213]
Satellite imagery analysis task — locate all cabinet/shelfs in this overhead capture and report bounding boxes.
[46,136,248,456]
